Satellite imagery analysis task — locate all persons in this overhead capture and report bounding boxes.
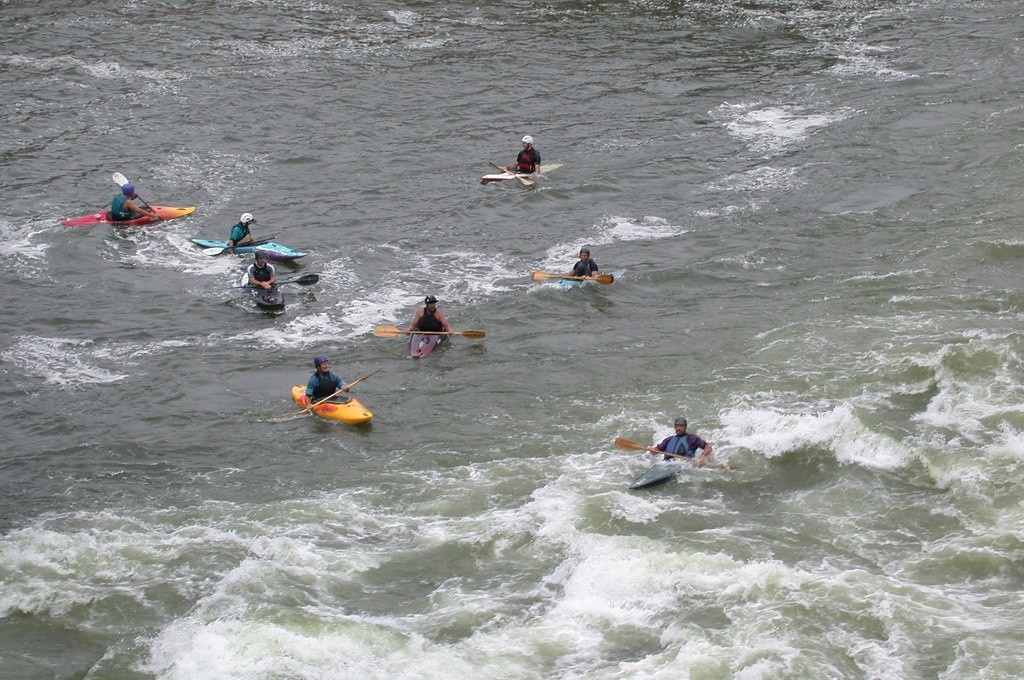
[560,248,598,280]
[247,251,277,289]
[650,417,713,466]
[403,294,455,336]
[229,213,257,255]
[500,135,542,176]
[112,183,160,220]
[305,355,349,412]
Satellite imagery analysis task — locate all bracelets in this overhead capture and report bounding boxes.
[447,328,452,332]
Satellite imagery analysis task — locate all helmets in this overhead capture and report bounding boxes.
[240,213,254,224]
[121,184,135,196]
[255,250,266,260]
[580,248,590,258]
[522,135,534,145]
[314,355,329,368]
[674,417,688,429]
[425,295,438,304]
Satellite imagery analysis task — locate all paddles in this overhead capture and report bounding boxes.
[615,437,704,464]
[372,324,486,338]
[294,367,383,416]
[532,269,614,285]
[488,161,534,186]
[202,235,277,257]
[235,273,319,286]
[112,171,167,223]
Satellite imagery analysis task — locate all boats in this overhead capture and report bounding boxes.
[409,327,447,358]
[558,268,628,290]
[291,385,374,424]
[629,464,682,489]
[191,238,308,261]
[241,273,286,310]
[61,205,196,227]
[482,163,565,181]
